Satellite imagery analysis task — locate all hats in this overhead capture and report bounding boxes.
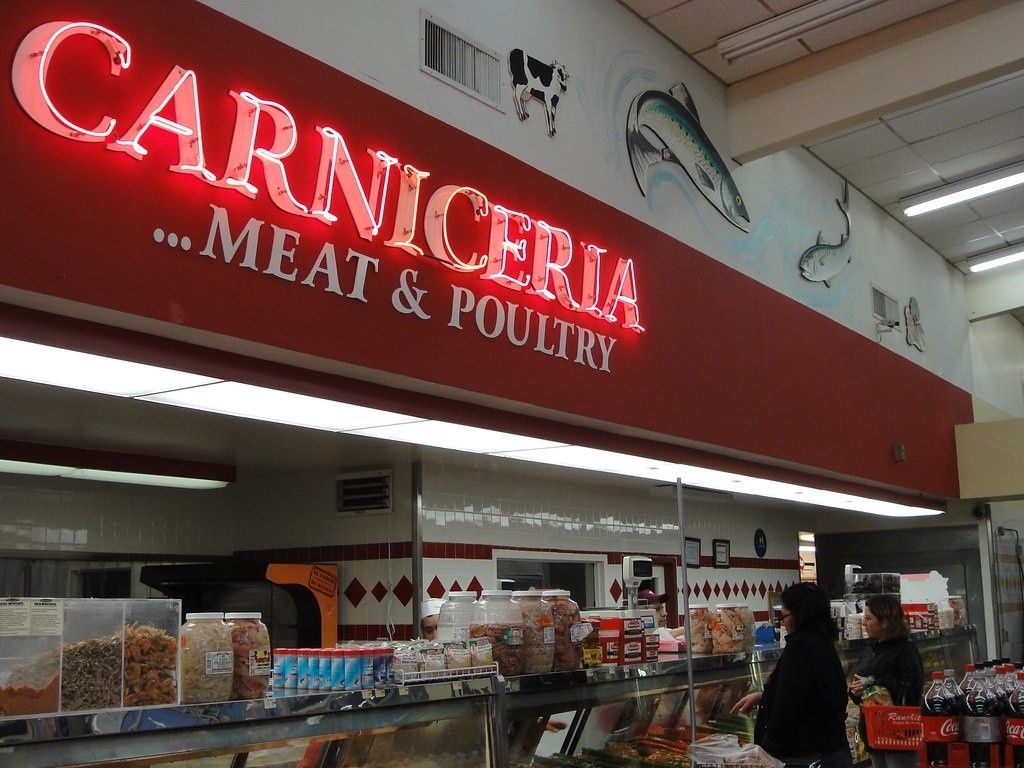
[421,598,447,619]
[638,589,670,605]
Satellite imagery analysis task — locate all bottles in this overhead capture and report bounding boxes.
[921,657,1024,768]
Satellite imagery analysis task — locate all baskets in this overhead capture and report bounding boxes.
[860,681,921,751]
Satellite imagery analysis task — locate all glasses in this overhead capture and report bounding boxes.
[774,613,793,627]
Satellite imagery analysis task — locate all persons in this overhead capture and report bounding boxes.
[848,594,925,768]
[420,598,567,752]
[729,582,853,768]
[637,589,686,727]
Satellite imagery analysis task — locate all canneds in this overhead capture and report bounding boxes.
[273,647,395,692]
